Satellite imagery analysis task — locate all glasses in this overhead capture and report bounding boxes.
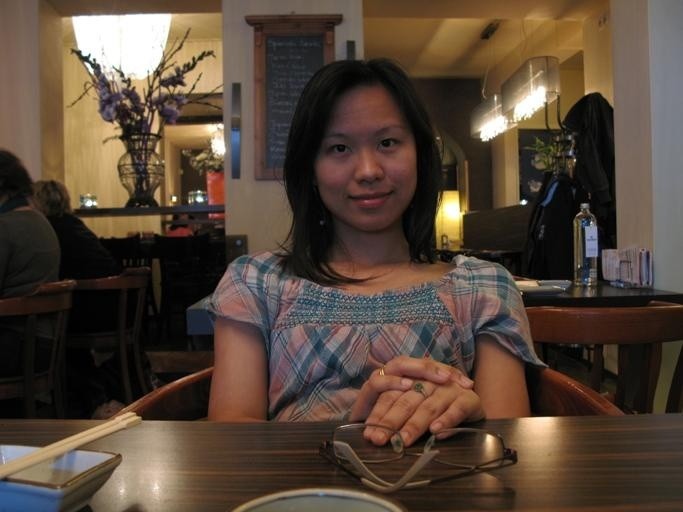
[318,423,517,494]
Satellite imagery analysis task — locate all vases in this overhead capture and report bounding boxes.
[115,133,166,211]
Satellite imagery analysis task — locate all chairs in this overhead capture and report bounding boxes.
[522,300,682,412]
[105,364,627,422]
[0,235,156,420]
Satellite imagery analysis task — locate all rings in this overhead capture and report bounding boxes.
[413,381,429,397]
[380,365,387,377]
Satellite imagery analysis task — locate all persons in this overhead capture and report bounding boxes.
[0,148,62,378]
[167,213,195,238]
[206,58,550,449]
[30,179,118,371]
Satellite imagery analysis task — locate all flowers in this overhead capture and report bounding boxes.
[62,28,224,194]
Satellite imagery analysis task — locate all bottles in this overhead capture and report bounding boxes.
[574,203,599,287]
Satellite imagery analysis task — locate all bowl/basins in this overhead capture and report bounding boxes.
[0,439,122,512]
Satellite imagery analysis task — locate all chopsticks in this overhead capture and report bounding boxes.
[0,410,143,480]
